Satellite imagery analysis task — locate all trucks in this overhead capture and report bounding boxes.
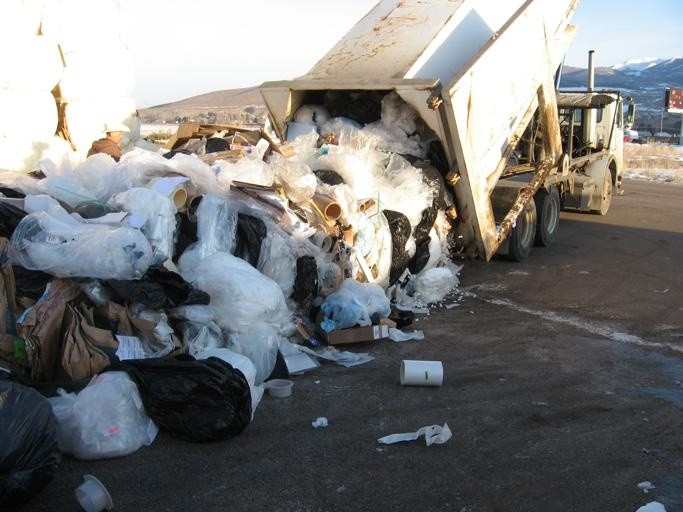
[256,0,635,264]
